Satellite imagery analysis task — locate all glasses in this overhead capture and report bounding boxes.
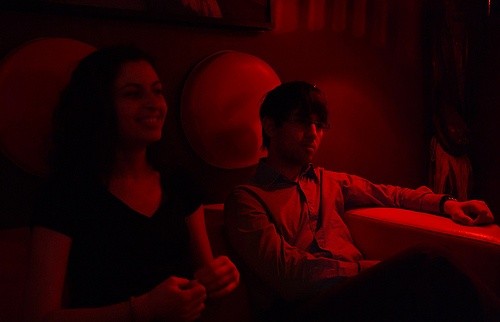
[286,118,330,130]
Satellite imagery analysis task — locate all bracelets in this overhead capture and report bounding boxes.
[223,82,495,322]
[128,296,140,322]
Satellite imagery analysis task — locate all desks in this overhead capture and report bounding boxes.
[344,207,500,322]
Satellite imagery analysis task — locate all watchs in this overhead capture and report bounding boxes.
[439,196,457,218]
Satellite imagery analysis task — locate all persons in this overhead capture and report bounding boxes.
[21,46,242,322]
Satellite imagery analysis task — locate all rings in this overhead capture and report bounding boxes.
[193,295,200,307]
